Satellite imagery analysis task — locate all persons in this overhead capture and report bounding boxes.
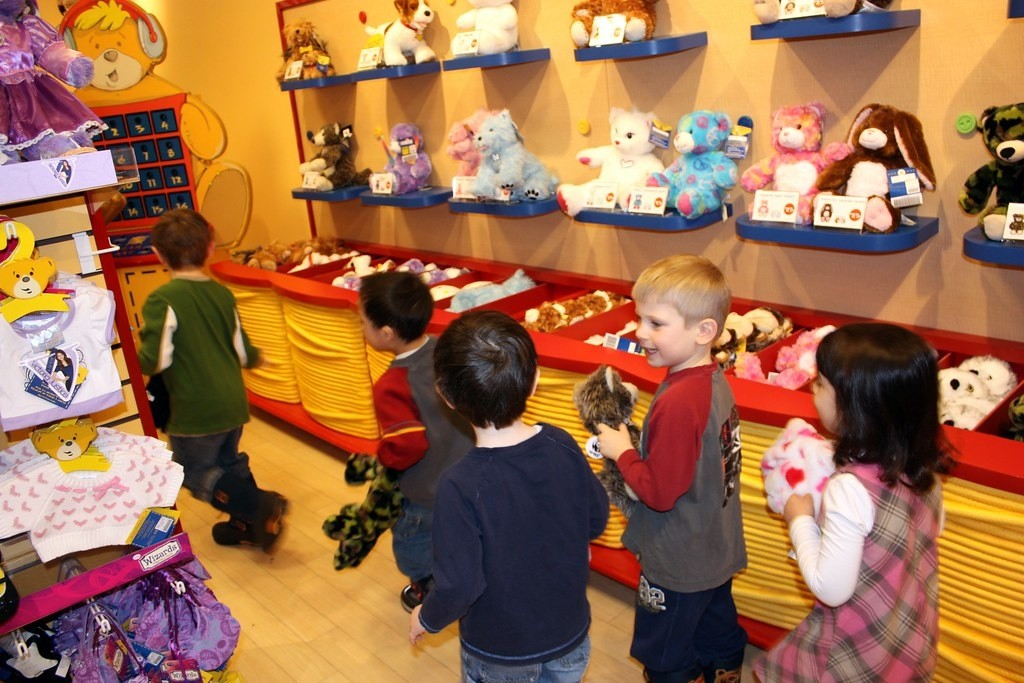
[137,208,285,551]
[411,309,609,683]
[598,255,748,683]
[359,271,476,613]
[752,322,954,683]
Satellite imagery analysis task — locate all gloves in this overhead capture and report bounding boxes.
[322,472,407,570]
[346,451,384,484]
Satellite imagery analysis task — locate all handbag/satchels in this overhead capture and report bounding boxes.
[53,567,241,683]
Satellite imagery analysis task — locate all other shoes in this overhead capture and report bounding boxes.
[402,584,421,612]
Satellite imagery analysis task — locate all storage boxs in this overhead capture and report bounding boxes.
[0,148,119,207]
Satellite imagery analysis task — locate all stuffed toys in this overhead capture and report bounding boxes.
[937,354,1024,443]
[711,307,835,390]
[753,0,857,24]
[578,367,642,519]
[457,0,518,54]
[231,236,629,332]
[147,374,169,428]
[761,418,842,519]
[323,452,408,570]
[299,103,937,232]
[959,101,1024,240]
[367,0,437,66]
[570,0,659,48]
[0,0,110,164]
[275,19,335,82]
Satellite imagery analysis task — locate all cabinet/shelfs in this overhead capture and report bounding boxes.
[279,0,1024,275]
[0,190,194,645]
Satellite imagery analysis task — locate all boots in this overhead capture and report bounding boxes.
[210,475,286,551]
[213,453,262,547]
